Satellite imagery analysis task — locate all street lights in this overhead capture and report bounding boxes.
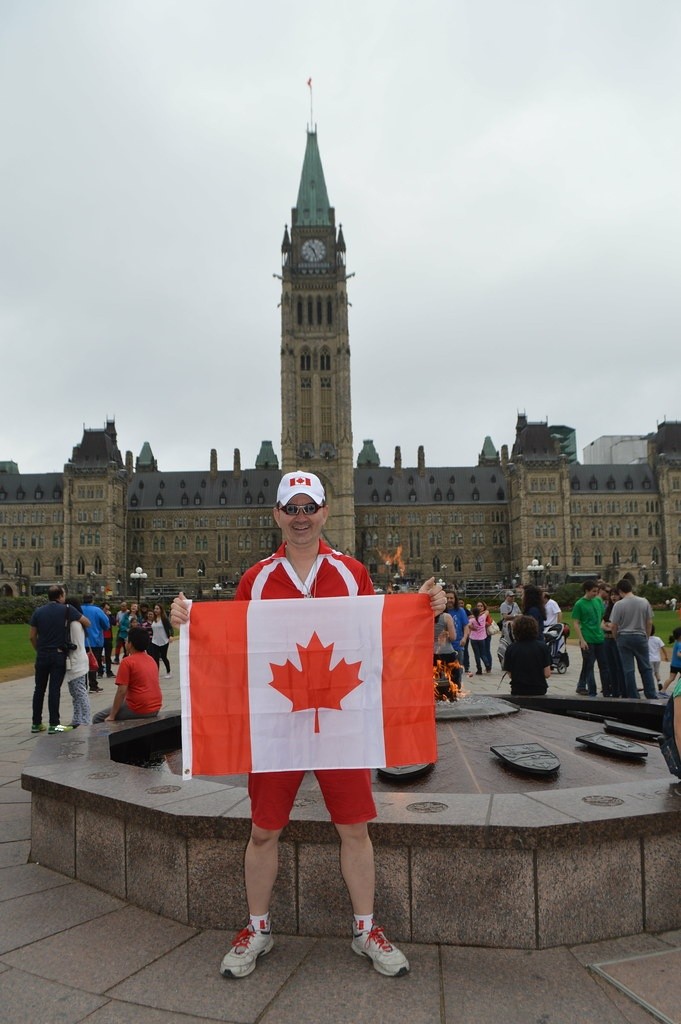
[527,559,545,588]
[441,563,448,581]
[197,568,203,599]
[383,561,393,586]
[129,566,148,612]
[650,560,657,583]
[212,583,223,602]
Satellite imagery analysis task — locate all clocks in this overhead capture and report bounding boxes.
[300,237,327,262]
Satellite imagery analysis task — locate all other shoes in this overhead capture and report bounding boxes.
[658,691,670,698]
[576,688,588,695]
[165,673,172,679]
[486,666,491,673]
[98,673,103,679]
[637,687,644,692]
[111,658,119,664]
[476,668,482,675]
[106,672,115,678]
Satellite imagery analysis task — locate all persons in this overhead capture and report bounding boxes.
[151,604,174,678]
[64,596,90,728]
[520,584,547,641]
[647,625,668,691]
[672,675,681,756]
[608,580,658,699]
[501,616,551,695]
[543,591,561,627]
[170,469,448,977]
[114,602,154,664]
[571,579,605,696]
[596,572,636,696]
[499,591,522,620]
[658,627,681,698]
[91,627,163,723]
[98,602,116,678]
[29,586,90,733]
[432,591,492,691]
[80,594,110,693]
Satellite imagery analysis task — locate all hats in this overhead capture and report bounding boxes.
[276,470,326,507]
[505,591,515,597]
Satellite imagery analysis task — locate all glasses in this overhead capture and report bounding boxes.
[281,502,322,516]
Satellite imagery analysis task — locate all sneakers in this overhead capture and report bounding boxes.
[351,921,410,976]
[31,724,47,731]
[89,685,104,693]
[48,724,73,734]
[219,923,274,978]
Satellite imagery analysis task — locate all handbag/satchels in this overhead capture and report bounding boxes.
[86,651,98,672]
[486,620,500,635]
[497,620,503,631]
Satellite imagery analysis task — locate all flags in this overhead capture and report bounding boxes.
[179,593,439,779]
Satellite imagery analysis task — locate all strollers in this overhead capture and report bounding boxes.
[544,621,570,674]
